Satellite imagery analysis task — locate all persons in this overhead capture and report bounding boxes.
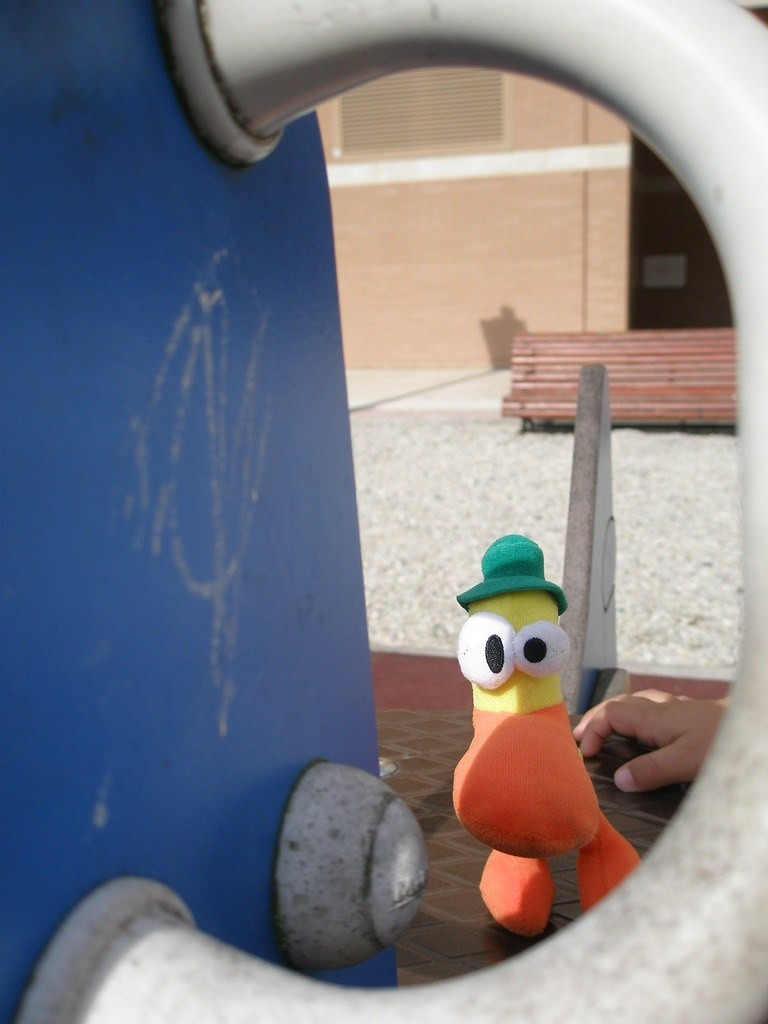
[570,688,726,792]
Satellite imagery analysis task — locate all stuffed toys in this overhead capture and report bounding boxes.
[452,535,641,938]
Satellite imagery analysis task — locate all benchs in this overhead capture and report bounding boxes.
[501,328,739,434]
